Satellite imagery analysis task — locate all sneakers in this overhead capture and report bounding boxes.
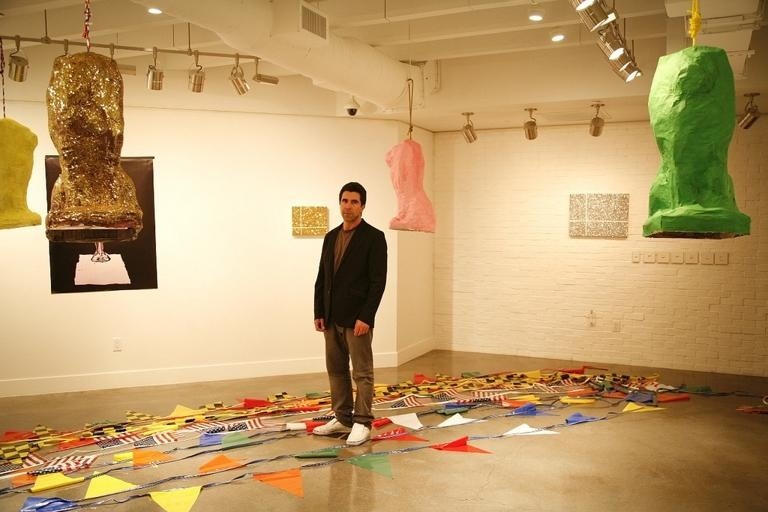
[346,423,372,446]
[313,418,352,435]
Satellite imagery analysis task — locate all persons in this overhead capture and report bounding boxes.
[313,181,388,446]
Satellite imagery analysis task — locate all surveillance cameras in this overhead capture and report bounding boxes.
[344,104,359,117]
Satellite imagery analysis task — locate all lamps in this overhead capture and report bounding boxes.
[737,92,760,130]
[588,103,605,137]
[461,112,478,145]
[528,0,643,84]
[0,9,279,95]
[523,108,538,140]
